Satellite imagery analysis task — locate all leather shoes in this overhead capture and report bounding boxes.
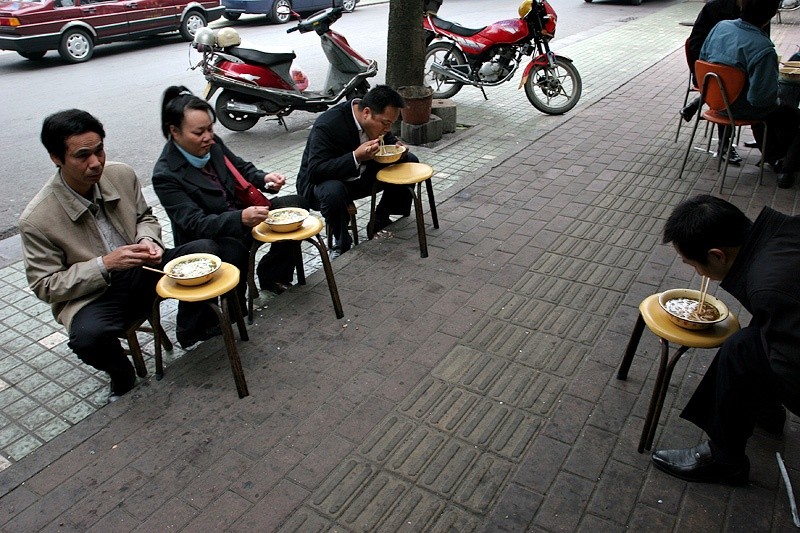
[258,275,292,294]
[651,439,751,487]
[679,99,697,122]
[717,146,742,163]
[754,403,786,431]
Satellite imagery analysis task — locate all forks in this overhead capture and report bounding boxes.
[696,275,710,314]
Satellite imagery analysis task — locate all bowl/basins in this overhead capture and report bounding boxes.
[658,288,729,330]
[373,145,406,163]
[264,207,309,232]
[163,253,222,286]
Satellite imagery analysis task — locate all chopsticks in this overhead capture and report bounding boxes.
[254,207,274,220]
[142,266,183,276]
[364,135,385,152]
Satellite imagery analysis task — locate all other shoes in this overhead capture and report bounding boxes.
[777,174,792,188]
[330,241,351,262]
[366,219,394,239]
[108,381,122,402]
[744,139,758,147]
[180,325,223,349]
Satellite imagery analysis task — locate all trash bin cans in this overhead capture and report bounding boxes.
[397,85,433,124]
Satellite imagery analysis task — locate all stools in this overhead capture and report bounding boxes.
[152,262,252,400]
[118,311,174,376]
[248,215,344,325]
[616,293,742,456]
[368,162,440,258]
[325,198,359,250]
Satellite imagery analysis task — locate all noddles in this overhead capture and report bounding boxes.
[266,210,304,223]
[379,136,385,155]
[665,298,720,322]
[169,258,217,278]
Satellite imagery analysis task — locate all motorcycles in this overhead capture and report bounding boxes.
[422,0,582,114]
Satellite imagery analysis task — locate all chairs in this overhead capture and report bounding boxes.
[678,59,768,195]
[675,70,742,153]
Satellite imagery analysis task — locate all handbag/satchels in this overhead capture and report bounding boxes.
[222,156,272,211]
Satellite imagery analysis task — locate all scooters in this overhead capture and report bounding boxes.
[190,4,380,129]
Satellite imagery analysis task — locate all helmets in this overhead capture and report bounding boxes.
[217,27,241,48]
[193,26,215,46]
[518,0,535,19]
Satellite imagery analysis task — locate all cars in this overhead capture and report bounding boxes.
[0,0,226,64]
[221,0,361,24]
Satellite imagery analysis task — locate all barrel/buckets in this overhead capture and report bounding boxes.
[397,85,435,125]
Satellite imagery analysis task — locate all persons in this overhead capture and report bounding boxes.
[651,194,800,487]
[296,84,420,262]
[679,0,800,189]
[152,85,310,323]
[19,109,223,404]
